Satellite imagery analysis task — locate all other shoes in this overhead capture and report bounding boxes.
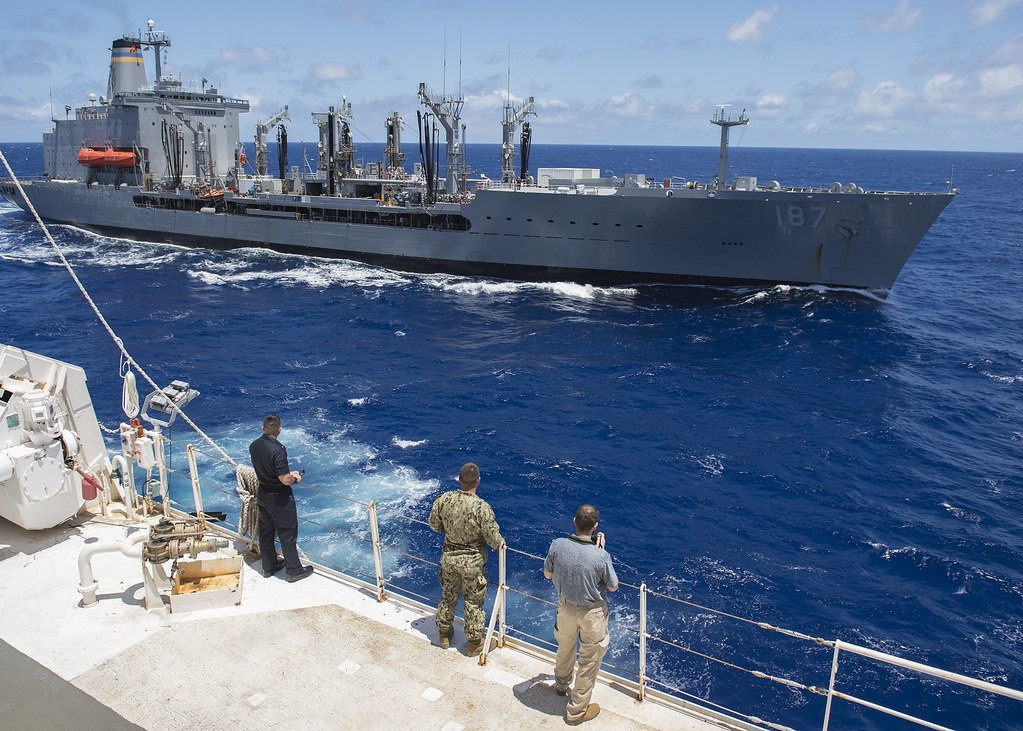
[440,626,455,649]
[467,638,497,657]
[288,565,313,583]
[264,559,285,578]
[557,690,567,696]
[566,703,600,725]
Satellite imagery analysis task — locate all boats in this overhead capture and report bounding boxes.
[200,188,235,199]
[76,147,137,167]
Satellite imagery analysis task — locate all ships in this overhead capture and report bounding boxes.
[0,18,957,300]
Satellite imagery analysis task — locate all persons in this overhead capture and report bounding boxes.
[544,505,619,726]
[249,415,313,583]
[511,176,522,190]
[436,191,471,203]
[428,462,505,657]
